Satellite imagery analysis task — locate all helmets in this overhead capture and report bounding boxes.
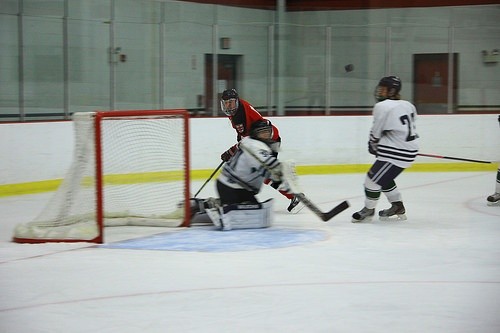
[374,75,402,102]
[219,88,239,116]
[248,119,272,141]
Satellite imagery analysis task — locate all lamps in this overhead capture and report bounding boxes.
[107,47,122,63]
[481,49,500,63]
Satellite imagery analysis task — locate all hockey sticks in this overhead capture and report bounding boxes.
[238,142,349,222]
[417,153,492,164]
[194,160,225,198]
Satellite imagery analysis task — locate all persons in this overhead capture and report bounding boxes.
[190,119,302,229]
[352,76,420,223]
[486,114,500,206]
[221,89,307,213]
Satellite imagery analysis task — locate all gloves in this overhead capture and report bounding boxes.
[220,152,231,162]
[368,141,377,156]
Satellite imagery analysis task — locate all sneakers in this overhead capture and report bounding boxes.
[287,193,305,214]
[204,207,222,229]
[487,193,500,206]
[379,200,407,221]
[352,207,375,223]
[189,198,197,220]
